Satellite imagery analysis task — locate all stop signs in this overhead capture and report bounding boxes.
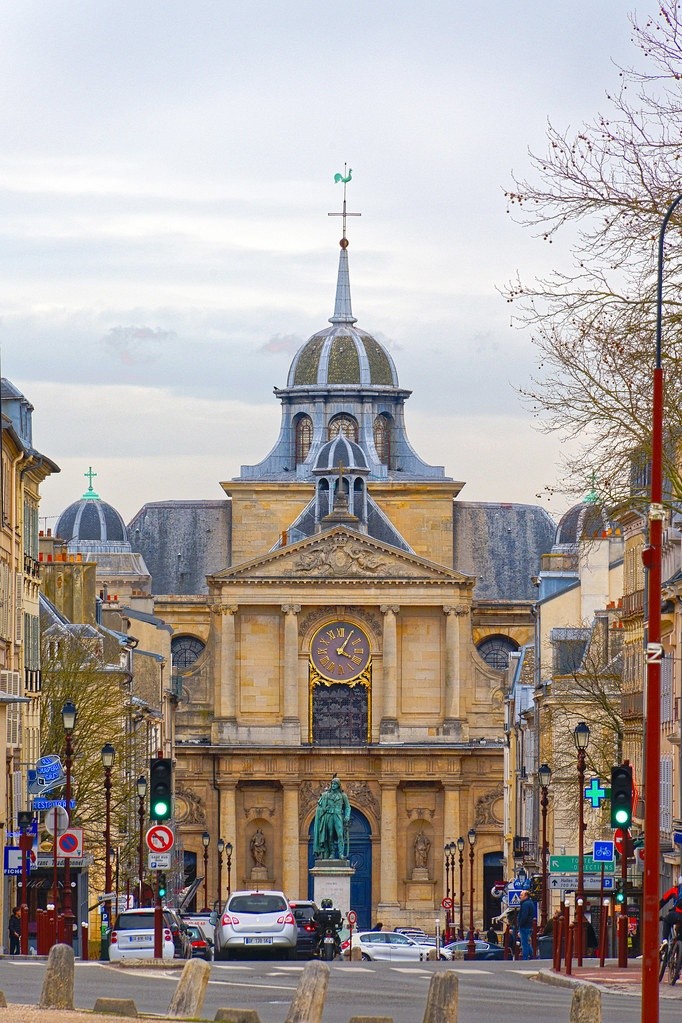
[614,828,634,858]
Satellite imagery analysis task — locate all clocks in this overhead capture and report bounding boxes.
[309,620,372,684]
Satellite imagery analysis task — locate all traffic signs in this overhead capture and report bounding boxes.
[110,905,126,912]
[110,896,127,903]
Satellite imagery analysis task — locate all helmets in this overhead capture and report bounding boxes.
[321,899,333,908]
[678,876,682,884]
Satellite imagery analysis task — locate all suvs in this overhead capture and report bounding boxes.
[277,899,319,960]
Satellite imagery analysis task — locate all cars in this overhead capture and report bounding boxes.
[338,930,453,961]
[105,907,221,961]
[209,891,304,962]
[392,927,513,960]
[535,905,640,959]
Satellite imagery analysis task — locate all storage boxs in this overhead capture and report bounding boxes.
[315,908,341,925]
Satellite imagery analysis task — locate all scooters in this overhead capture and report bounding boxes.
[308,921,340,961]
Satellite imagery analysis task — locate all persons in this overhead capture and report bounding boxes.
[487,927,499,944]
[466,927,480,939]
[313,778,351,859]
[517,891,535,959]
[659,876,682,976]
[372,923,383,931]
[310,899,344,960]
[9,907,20,955]
[414,831,431,867]
[251,829,265,866]
[509,929,521,949]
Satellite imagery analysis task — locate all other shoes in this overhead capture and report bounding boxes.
[660,941,668,951]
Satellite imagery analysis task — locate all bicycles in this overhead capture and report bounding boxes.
[657,916,682,987]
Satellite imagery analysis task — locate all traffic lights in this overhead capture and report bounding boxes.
[615,881,625,904]
[611,765,633,828]
[156,873,167,899]
[150,757,174,821]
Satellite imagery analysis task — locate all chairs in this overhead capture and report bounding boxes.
[266,898,280,913]
[234,899,249,913]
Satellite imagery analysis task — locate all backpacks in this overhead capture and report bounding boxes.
[673,884,682,909]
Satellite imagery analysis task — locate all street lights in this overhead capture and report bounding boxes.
[202,831,211,911]
[467,828,477,961]
[134,775,149,908]
[59,698,79,947]
[217,838,225,914]
[443,843,451,943]
[457,836,466,941]
[536,763,553,932]
[572,721,591,957]
[225,843,233,898]
[100,742,117,929]
[450,841,456,942]
